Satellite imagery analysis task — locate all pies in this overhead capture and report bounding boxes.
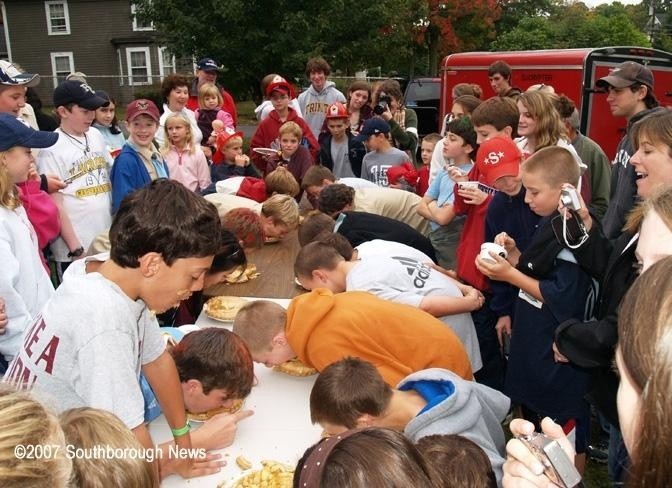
[282,359,316,377]
[227,262,260,282]
[188,400,241,419]
[206,297,247,319]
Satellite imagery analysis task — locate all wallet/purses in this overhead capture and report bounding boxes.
[550,209,590,250]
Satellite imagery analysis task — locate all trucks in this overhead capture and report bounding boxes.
[437,45,672,170]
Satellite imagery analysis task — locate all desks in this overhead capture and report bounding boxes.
[201,175,312,301]
[139,294,326,488]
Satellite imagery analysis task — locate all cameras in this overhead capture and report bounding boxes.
[374,96,391,114]
[562,189,582,211]
[517,430,583,488]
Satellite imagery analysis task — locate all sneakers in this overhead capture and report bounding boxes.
[588,434,612,464]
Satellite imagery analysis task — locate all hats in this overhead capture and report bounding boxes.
[125,98,159,123]
[53,80,109,111]
[266,74,294,99]
[0,112,58,152]
[595,61,654,91]
[196,57,220,72]
[0,59,40,88]
[325,103,348,119]
[351,115,390,141]
[475,137,521,187]
[211,131,243,165]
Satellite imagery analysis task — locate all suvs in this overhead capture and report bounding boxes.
[399,75,441,153]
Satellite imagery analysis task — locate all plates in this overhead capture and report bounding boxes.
[253,147,279,155]
[203,296,247,321]
[191,399,247,425]
[295,276,311,291]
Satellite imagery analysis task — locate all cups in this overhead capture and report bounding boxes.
[479,241,508,263]
[458,181,479,192]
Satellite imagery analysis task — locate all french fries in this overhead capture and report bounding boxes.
[229,454,295,488]
